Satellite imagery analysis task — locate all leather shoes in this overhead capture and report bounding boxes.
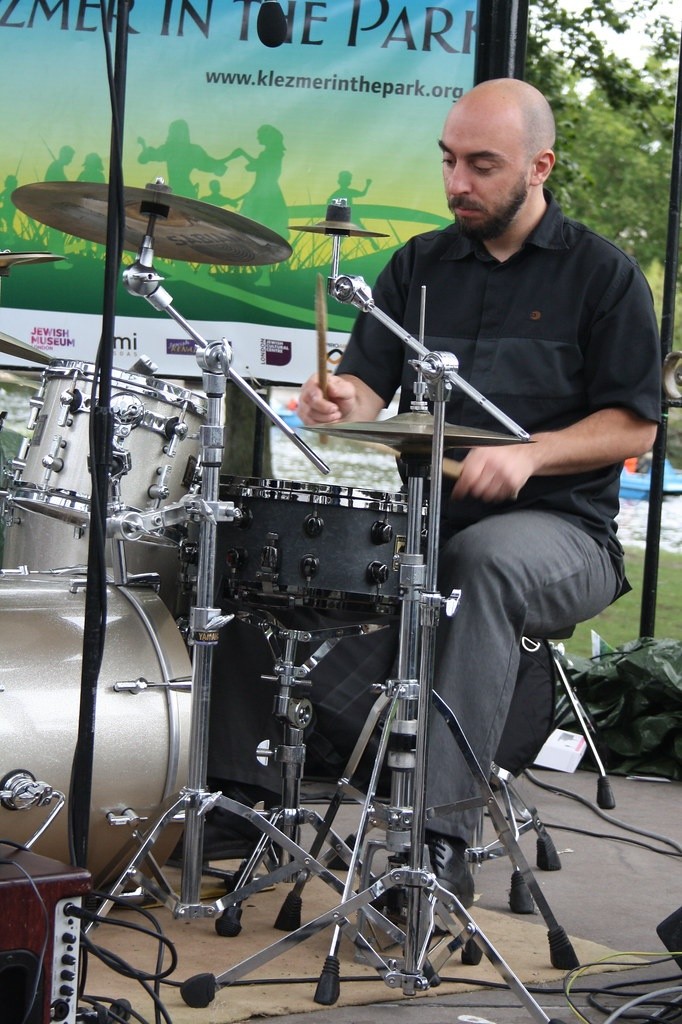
[173,785,300,861]
[369,839,475,909]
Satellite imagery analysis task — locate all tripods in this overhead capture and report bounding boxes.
[80,213,578,1024]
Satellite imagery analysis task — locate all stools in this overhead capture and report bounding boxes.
[522,626,616,811]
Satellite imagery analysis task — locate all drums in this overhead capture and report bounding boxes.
[2,500,185,619]
[7,359,209,550]
[0,563,202,903]
[222,477,429,623]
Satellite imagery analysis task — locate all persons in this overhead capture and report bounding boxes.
[167,79,663,916]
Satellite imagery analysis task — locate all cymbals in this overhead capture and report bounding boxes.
[0,250,66,271]
[291,218,390,238]
[301,412,535,450]
[11,180,293,265]
[1,330,52,365]
